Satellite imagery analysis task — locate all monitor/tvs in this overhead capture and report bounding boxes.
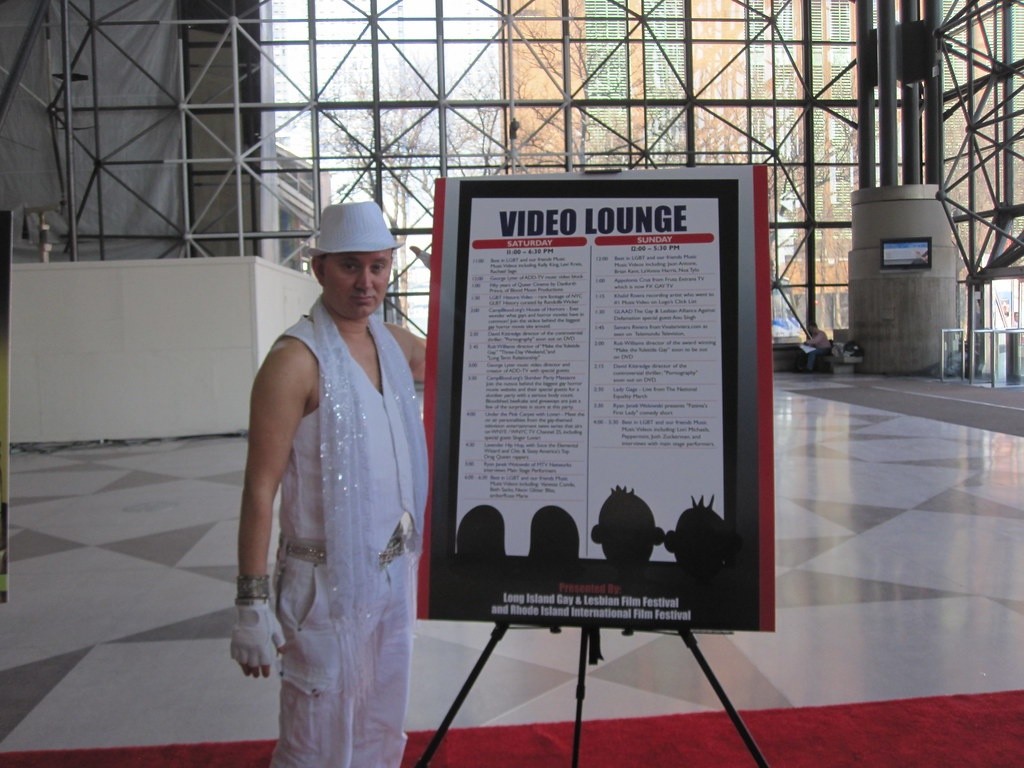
[880,237,932,270]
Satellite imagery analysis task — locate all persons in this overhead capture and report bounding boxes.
[795,324,832,373]
[232,203,427,768]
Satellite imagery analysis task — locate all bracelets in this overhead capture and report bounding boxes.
[237,575,270,599]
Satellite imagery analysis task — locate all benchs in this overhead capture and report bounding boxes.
[822,356,863,374]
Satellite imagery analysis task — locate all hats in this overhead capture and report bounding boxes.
[307,201,405,257]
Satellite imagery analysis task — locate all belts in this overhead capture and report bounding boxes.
[276,535,408,570]
